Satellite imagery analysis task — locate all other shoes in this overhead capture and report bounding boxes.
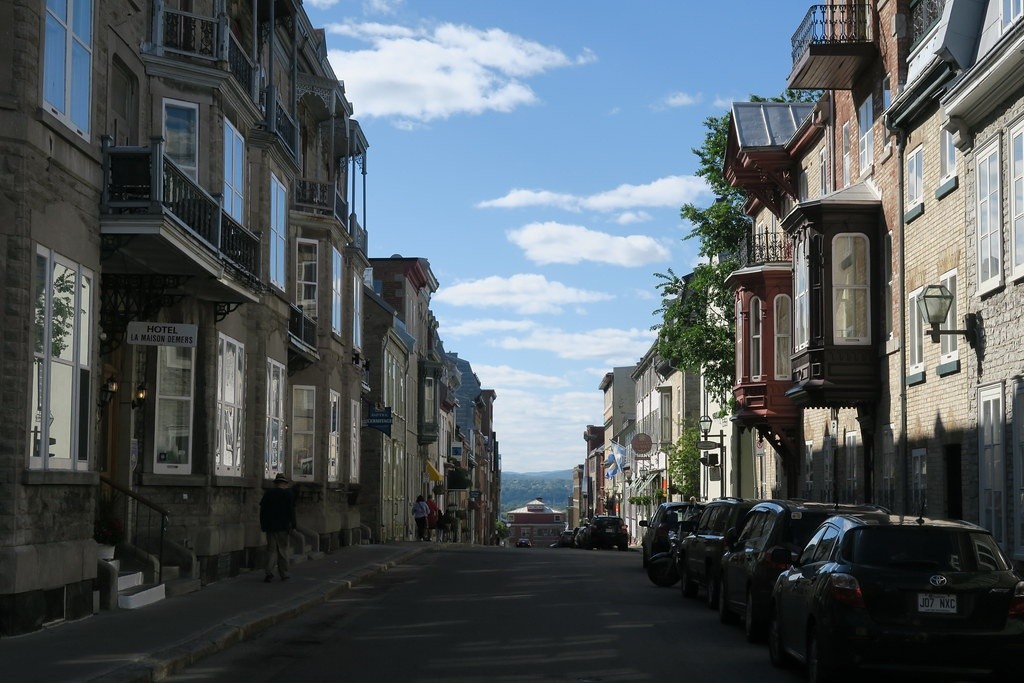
[426,537,432,542]
[280,576,293,584]
[416,537,424,542]
[264,576,273,583]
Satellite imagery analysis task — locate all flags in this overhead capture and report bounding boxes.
[604,460,618,480]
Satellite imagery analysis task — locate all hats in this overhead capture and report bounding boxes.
[272,473,289,483]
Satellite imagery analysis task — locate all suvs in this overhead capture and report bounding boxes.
[767,510,1023,683]
[639,502,706,568]
[676,494,772,603]
[719,500,896,643]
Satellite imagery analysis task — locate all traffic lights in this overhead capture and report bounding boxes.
[38,437,57,458]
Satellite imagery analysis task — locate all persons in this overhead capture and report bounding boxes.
[412,494,452,542]
[259,473,297,583]
[683,496,699,522]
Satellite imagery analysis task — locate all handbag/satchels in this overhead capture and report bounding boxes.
[425,518,429,529]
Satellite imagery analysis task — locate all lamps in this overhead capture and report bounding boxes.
[639,465,648,482]
[917,283,979,350]
[97,373,119,408]
[119,380,147,410]
[698,415,726,442]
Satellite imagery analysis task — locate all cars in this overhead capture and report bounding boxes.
[550,515,628,551]
[516,539,531,547]
